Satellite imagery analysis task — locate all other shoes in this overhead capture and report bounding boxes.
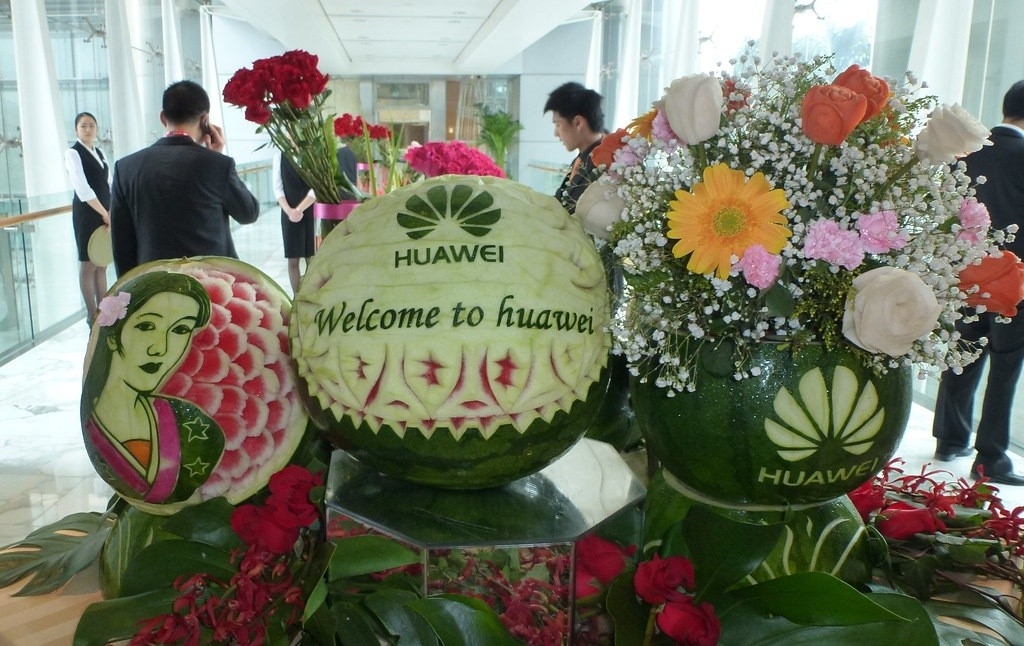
[970,462,1024,486]
[934,438,975,462]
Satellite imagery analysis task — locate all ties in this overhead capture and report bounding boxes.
[569,158,581,185]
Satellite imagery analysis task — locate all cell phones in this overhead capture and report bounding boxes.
[203,118,215,144]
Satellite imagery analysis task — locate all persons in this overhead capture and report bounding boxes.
[111,80,259,278]
[932,79,1024,488]
[542,84,631,318]
[272,116,321,298]
[313,120,363,249]
[65,112,113,333]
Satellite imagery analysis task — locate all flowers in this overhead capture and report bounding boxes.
[127,464,326,646]
[219,48,509,202]
[845,455,1024,584]
[93,291,132,327]
[563,533,724,646]
[556,34,1024,403]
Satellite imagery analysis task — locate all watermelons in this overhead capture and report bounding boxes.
[327,463,589,551]
[642,466,872,591]
[287,173,613,490]
[80,256,312,515]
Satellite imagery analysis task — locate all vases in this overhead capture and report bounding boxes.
[312,202,364,256]
